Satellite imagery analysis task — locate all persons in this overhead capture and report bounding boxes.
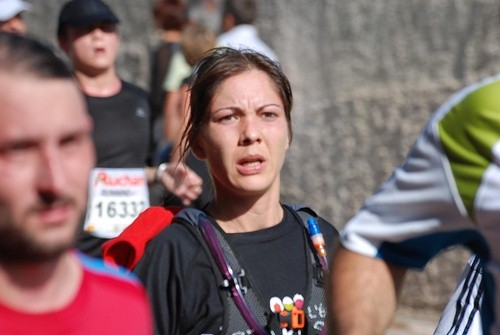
[326,69,500,335]
[131,46,343,334]
[0,0,277,262]
[2,33,154,335]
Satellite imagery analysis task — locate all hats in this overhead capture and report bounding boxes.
[58,0,117,25]
